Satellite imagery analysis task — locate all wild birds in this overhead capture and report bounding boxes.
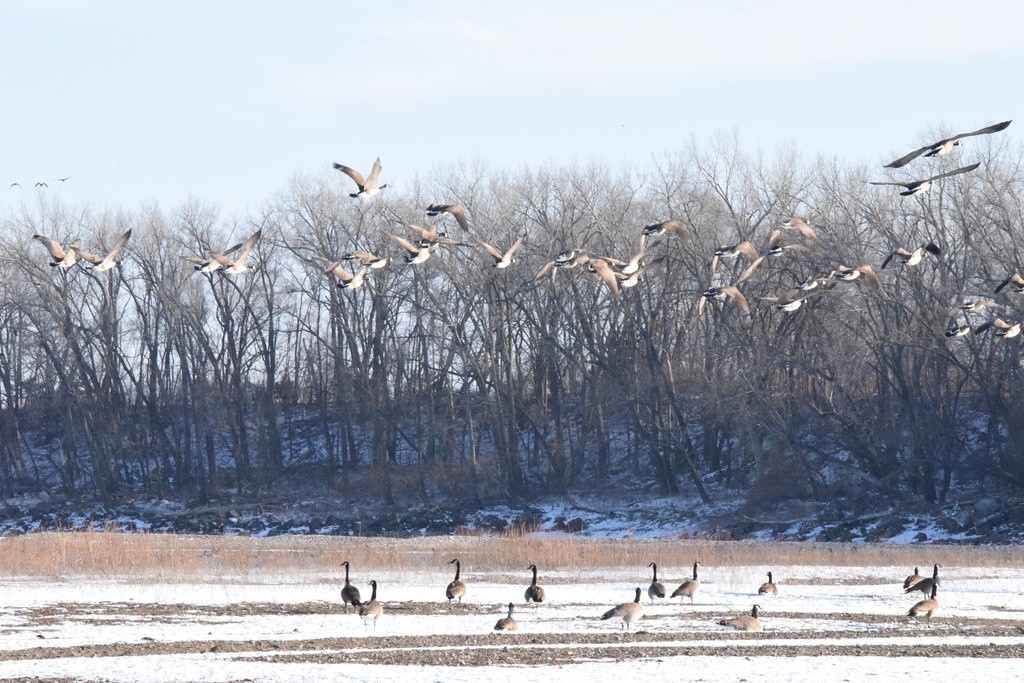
[717,605,762,632]
[671,562,701,605]
[332,155,393,199]
[524,565,545,603]
[882,241,1024,343]
[600,588,641,631]
[445,559,466,602]
[493,602,518,631]
[340,560,362,612]
[648,563,666,606]
[331,207,885,329]
[904,563,943,627]
[181,230,267,275]
[9,175,70,190]
[33,227,133,273]
[758,571,777,595]
[859,120,1013,196]
[359,579,384,633]
